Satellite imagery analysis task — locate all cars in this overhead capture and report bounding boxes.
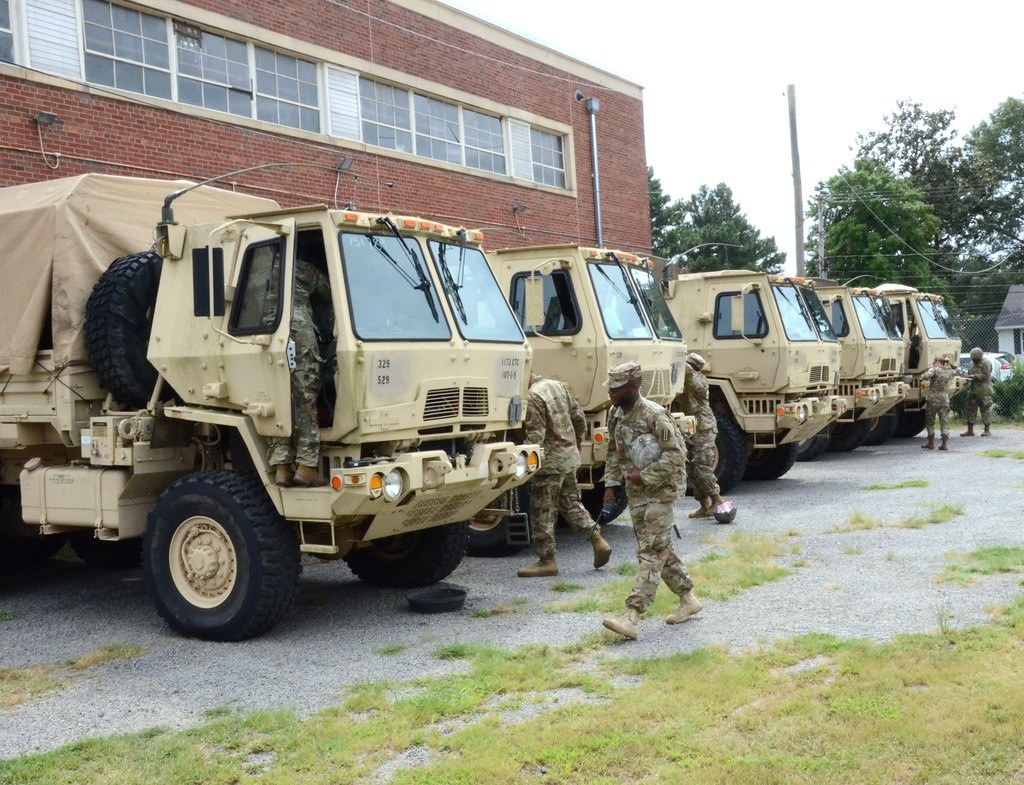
[960,351,1015,382]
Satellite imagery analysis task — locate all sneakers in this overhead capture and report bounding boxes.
[293,464,327,486]
[275,463,301,486]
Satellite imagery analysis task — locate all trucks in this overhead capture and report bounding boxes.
[482,245,687,555]
[877,284,962,438]
[805,277,910,453]
[655,269,845,498]
[0,167,547,641]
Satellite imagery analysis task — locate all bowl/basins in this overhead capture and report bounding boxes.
[406,589,467,613]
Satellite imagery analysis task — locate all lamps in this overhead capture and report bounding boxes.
[36,110,59,125]
[512,204,528,212]
[339,156,355,170]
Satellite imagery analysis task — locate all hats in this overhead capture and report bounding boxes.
[602,360,642,388]
[686,353,706,369]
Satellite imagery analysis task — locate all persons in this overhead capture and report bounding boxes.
[601,361,702,640]
[919,357,961,450]
[960,348,993,437]
[677,353,725,518]
[517,370,612,578]
[235,242,334,487]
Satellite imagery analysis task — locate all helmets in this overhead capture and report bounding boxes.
[713,508,736,523]
[629,433,662,470]
[970,347,982,358]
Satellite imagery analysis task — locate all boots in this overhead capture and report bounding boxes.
[960,423,974,437]
[981,425,990,436]
[938,438,948,450]
[921,436,935,448]
[666,589,702,624]
[518,554,558,577]
[603,607,640,640]
[689,498,711,518]
[705,494,724,516]
[591,530,612,568]
